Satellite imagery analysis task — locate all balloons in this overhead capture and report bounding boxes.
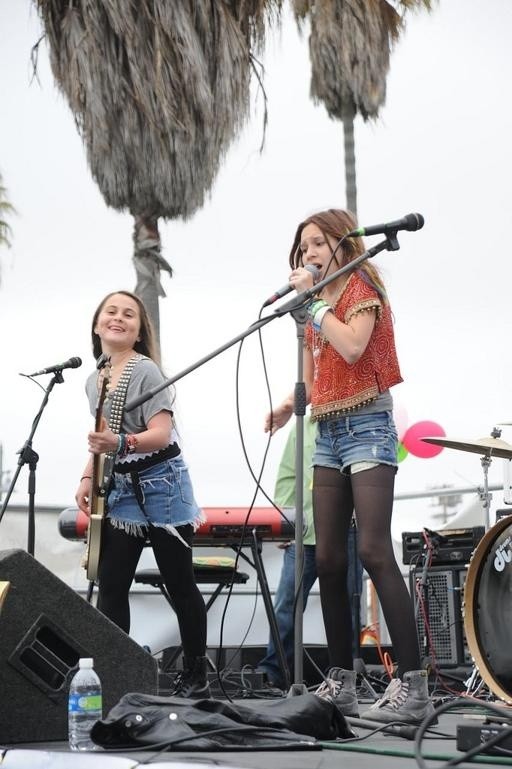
[397,441,408,464]
[401,421,446,458]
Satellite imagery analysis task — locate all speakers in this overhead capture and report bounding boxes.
[408,567,459,670]
[0,548,159,745]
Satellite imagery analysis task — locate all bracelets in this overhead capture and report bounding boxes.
[81,476,92,482]
[115,434,137,454]
[306,298,335,332]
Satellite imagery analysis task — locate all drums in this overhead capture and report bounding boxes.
[462,517,512,706]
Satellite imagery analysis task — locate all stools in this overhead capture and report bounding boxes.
[136,566,250,672]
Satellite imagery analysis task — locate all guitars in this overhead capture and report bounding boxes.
[85,358,115,582]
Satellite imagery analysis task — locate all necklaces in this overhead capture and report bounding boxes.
[311,329,324,382]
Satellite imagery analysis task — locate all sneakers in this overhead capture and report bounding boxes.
[312,666,362,719]
[169,655,211,700]
[361,670,440,729]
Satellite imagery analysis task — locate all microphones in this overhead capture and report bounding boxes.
[31,357,82,376]
[347,211,424,237]
[265,265,319,304]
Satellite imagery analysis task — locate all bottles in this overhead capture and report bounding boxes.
[67,656,102,752]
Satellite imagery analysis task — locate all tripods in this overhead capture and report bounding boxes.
[122,237,424,742]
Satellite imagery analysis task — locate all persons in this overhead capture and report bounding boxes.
[258,405,362,689]
[74,291,213,699]
[264,208,440,726]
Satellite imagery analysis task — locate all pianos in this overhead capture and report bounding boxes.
[61,508,298,540]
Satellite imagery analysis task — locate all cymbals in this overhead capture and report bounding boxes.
[421,434,511,459]
